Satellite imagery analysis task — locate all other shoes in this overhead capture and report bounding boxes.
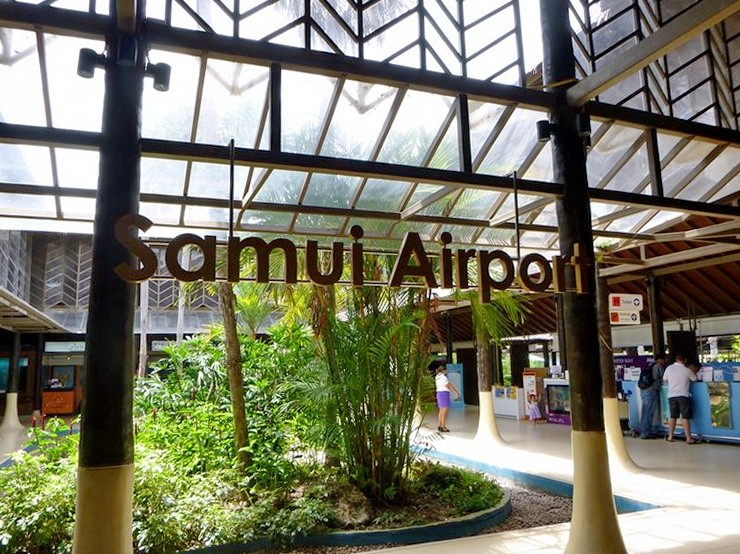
[688,438,701,444]
[437,426,450,432]
[664,435,673,442]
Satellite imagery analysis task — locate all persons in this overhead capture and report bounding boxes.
[638,353,704,444]
[525,392,542,423]
[434,366,461,431]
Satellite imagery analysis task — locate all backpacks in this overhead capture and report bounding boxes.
[638,362,657,389]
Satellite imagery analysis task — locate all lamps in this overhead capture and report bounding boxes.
[146,62,172,91]
[537,120,557,141]
[77,48,106,77]
[579,112,592,135]
